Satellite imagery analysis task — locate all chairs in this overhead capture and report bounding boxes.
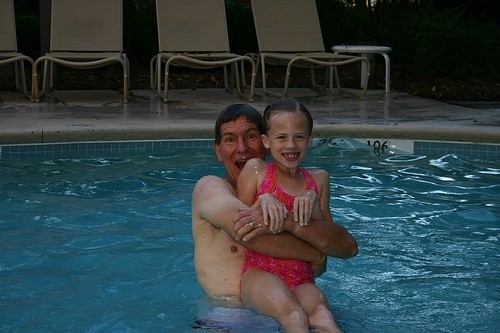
[244,0,369,104]
[149,0,258,103]
[32,0,131,106]
[0,0,35,105]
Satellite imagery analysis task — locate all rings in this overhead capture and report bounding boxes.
[249,222,254,230]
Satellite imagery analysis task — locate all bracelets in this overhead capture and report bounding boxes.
[312,253,327,265]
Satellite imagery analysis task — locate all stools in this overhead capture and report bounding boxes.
[330,43,392,94]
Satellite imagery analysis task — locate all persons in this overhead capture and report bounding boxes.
[237,99,342,333]
[192,104,358,333]
